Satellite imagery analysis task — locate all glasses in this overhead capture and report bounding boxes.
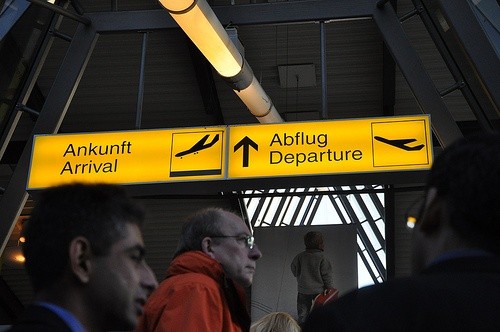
[212,234,254,249]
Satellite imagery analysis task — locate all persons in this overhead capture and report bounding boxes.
[0,182,304,332]
[290,231,334,324]
[301,133,500,332]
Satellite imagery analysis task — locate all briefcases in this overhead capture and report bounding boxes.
[313,287,340,306]
